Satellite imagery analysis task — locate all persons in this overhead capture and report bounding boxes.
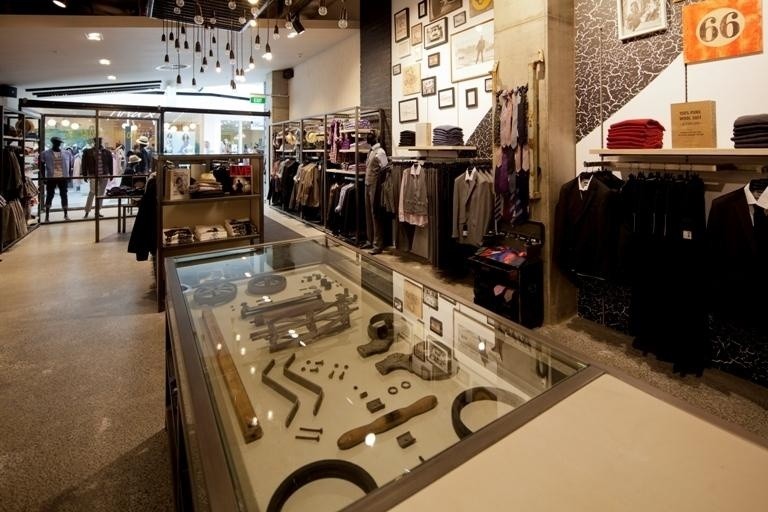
[39,137,74,223]
[120,156,142,190]
[359,126,388,255]
[82,137,113,217]
[136,136,154,172]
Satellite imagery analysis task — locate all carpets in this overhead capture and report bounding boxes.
[264,214,305,242]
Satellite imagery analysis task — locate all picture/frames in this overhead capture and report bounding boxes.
[438,87,455,108]
[453,10,466,28]
[397,40,411,59]
[401,63,421,96]
[616,0,668,41]
[421,76,436,96]
[450,18,495,83]
[394,7,410,43]
[485,78,492,92]
[418,0,427,19]
[411,22,422,46]
[424,17,448,49]
[428,52,440,67]
[414,45,423,62]
[466,88,478,107]
[399,97,418,123]
[392,64,401,75]
[468,0,494,18]
[429,0,462,22]
[682,1,763,65]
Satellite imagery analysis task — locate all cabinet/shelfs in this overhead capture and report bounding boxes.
[164,235,767,511]
[270,106,384,247]
[0,105,40,252]
[469,221,549,329]
[158,155,264,312]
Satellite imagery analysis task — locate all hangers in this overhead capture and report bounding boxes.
[468,157,493,171]
[580,160,694,182]
[386,160,446,193]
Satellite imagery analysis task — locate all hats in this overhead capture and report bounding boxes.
[50,137,64,142]
[127,155,143,163]
[306,130,319,144]
[135,136,149,145]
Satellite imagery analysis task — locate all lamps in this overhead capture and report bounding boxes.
[202,23,208,69]
[293,12,305,35]
[212,27,216,43]
[230,25,237,90]
[255,15,261,50]
[239,8,246,24]
[229,20,236,65]
[194,0,203,23]
[192,9,196,85]
[209,26,213,57]
[210,9,217,24]
[235,21,240,81]
[184,14,189,49]
[175,20,180,53]
[338,2,348,29]
[162,20,165,41]
[169,20,174,40]
[52,0,67,8]
[177,18,181,84]
[181,15,185,33]
[249,26,255,69]
[215,27,221,73]
[285,13,293,30]
[239,24,245,83]
[273,1,280,41]
[195,25,201,51]
[200,24,205,73]
[265,0,272,60]
[164,19,169,62]
[225,29,230,55]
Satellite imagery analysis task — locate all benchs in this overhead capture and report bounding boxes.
[123,204,140,233]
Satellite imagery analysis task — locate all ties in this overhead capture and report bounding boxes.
[500,94,505,146]
[495,148,521,223]
[520,146,529,221]
[504,289,516,302]
[511,89,518,151]
[517,87,528,143]
[505,93,511,148]
[493,284,504,297]
